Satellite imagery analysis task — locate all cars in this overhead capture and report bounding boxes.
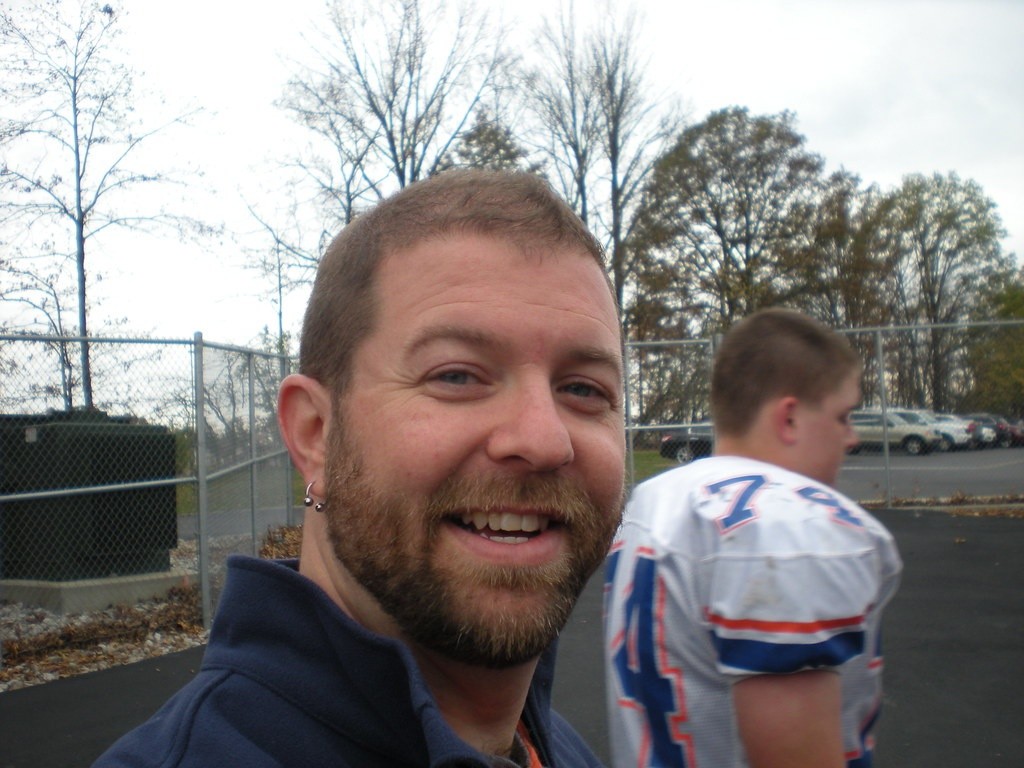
[659,419,714,465]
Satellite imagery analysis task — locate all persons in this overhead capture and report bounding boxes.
[599,307,902,768]
[92,170,626,768]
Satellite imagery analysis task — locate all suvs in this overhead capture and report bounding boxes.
[846,410,1024,458]
